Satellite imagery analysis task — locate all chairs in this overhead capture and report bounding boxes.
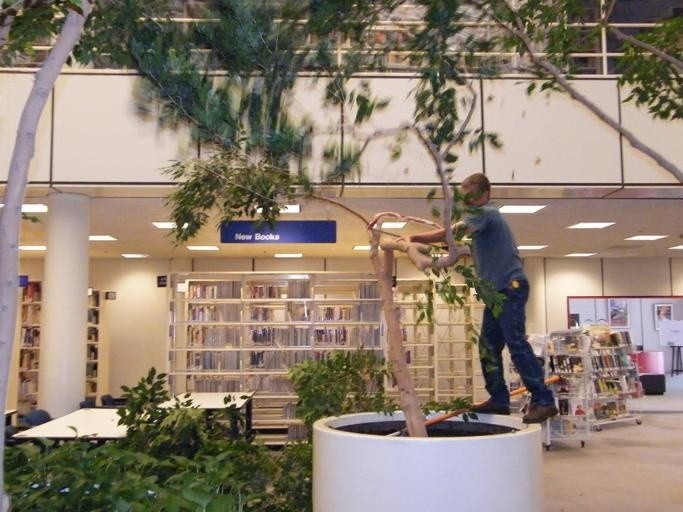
[4,408,50,436]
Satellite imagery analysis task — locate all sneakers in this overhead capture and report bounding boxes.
[468,398,512,416]
[522,402,559,423]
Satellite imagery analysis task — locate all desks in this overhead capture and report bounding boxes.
[11,404,155,439]
[155,390,258,433]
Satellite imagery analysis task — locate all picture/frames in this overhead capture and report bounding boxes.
[652,302,675,332]
[606,298,630,328]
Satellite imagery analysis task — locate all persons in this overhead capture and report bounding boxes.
[395,172,556,424]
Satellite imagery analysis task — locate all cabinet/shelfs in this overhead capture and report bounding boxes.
[85,286,111,406]
[17,279,44,408]
[530,323,645,451]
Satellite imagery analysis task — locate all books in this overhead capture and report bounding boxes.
[546,331,633,422]
